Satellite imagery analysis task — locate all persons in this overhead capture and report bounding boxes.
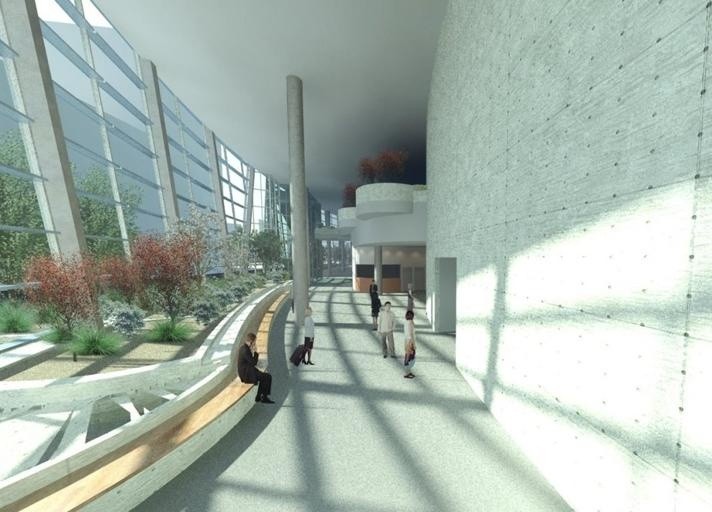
[237,333,276,403]
[288,282,296,313]
[369,278,417,379]
[301,307,317,366]
